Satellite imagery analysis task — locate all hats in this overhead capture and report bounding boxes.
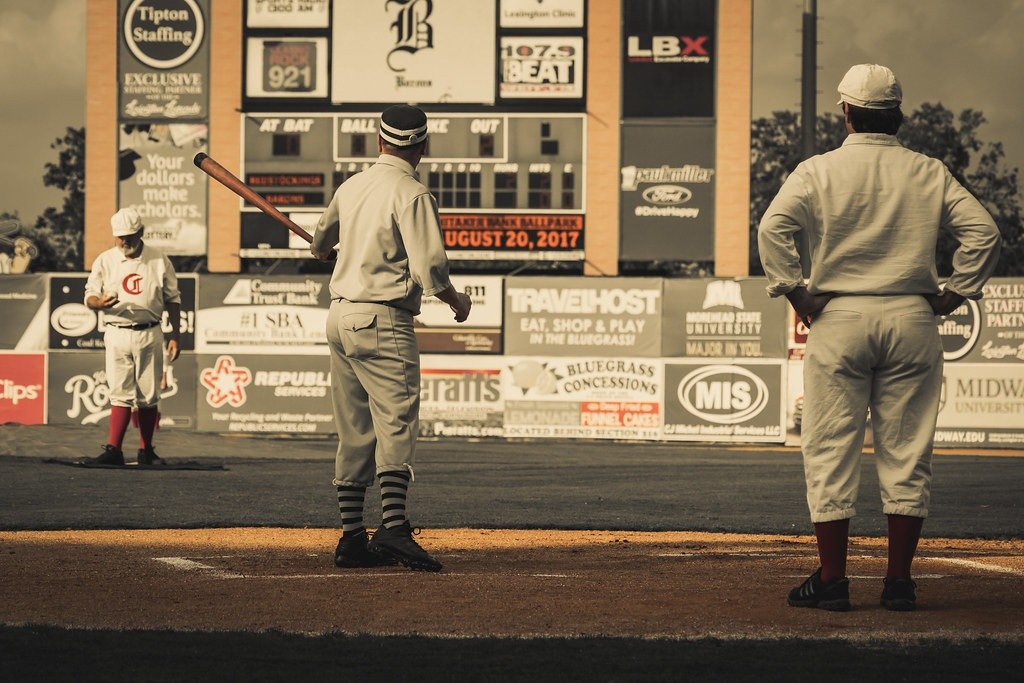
[837,63,903,109]
[379,105,428,149]
[111,208,143,236]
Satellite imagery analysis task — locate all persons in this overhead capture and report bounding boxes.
[309,106,472,572]
[71,207,182,469]
[752,63,1001,610]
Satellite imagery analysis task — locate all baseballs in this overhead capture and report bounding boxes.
[107,291,118,299]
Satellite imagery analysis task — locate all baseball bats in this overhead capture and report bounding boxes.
[194,152,337,261]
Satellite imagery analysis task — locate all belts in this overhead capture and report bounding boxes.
[106,321,160,331]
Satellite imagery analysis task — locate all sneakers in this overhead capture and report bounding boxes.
[786,568,851,611]
[879,578,918,610]
[334,531,399,567]
[367,520,443,572]
[84,444,124,468]
[137,447,165,466]
